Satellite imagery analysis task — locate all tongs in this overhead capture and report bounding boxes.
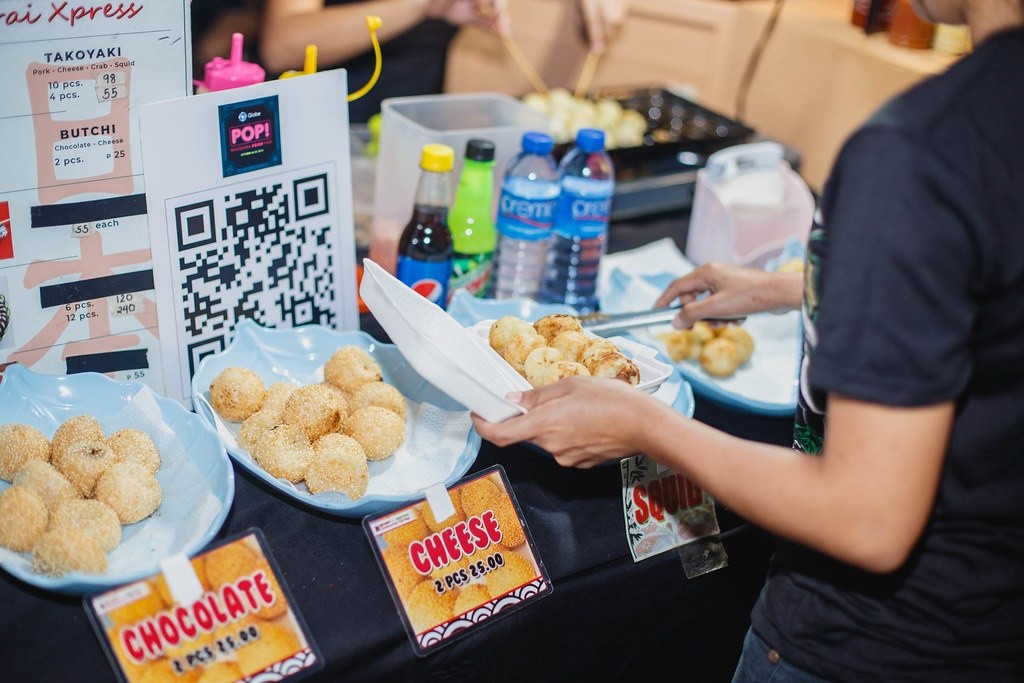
[569,301,746,336]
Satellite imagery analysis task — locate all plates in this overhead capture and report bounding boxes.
[0,363,235,594]
[447,287,695,418]
[600,236,813,417]
[191,318,481,516]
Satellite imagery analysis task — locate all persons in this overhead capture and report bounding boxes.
[471,0,1024,683]
[189,0,630,119]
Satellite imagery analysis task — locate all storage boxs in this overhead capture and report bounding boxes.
[371,92,547,246]
[550,88,757,221]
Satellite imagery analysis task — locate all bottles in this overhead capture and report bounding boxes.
[394,128,615,315]
[196,31,264,94]
[278,14,382,102]
